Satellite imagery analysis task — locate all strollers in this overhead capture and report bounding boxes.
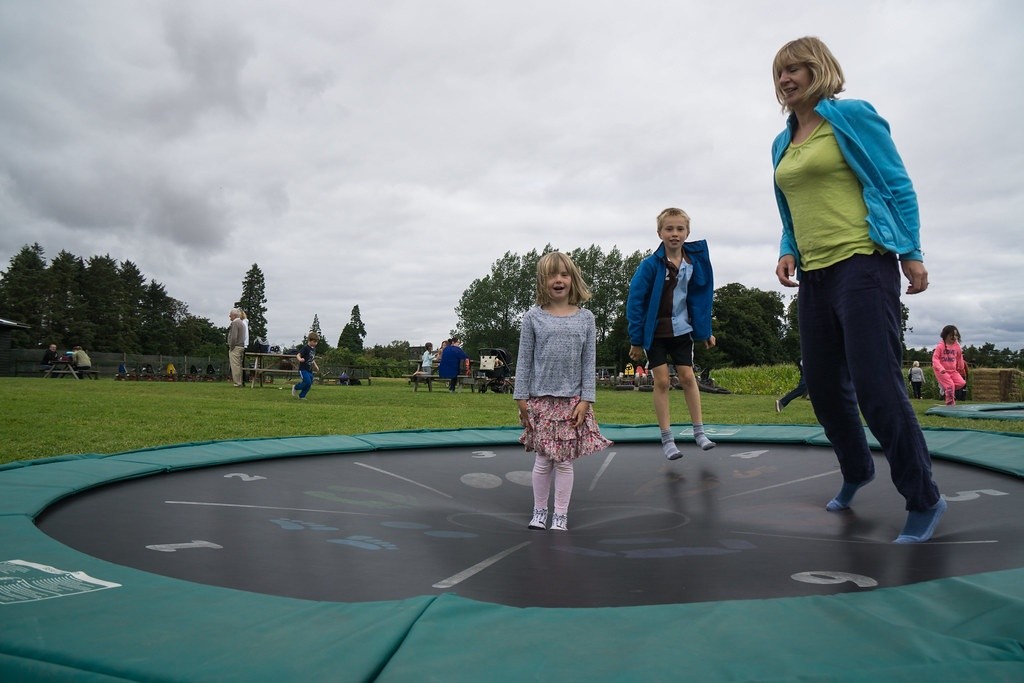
[475,347,515,395]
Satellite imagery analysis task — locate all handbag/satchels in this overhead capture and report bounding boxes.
[908,369,912,381]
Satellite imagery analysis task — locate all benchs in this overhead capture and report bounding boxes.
[77,369,99,373]
[402,373,469,379]
[243,367,317,378]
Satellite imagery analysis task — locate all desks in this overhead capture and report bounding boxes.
[43,360,92,381]
[245,352,322,390]
[408,359,480,394]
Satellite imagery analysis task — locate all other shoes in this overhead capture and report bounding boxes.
[299,397,307,401]
[292,385,297,397]
[775,400,784,412]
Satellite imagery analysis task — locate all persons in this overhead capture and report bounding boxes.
[775,358,808,413]
[908,361,926,399]
[932,326,966,407]
[771,36,947,546]
[955,360,968,401]
[39,344,62,378]
[627,208,716,460]
[414,337,470,393]
[292,332,320,400]
[226,309,249,386]
[71,345,91,380]
[512,253,614,531]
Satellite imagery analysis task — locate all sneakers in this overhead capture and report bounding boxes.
[528,506,548,529]
[551,513,568,531]
